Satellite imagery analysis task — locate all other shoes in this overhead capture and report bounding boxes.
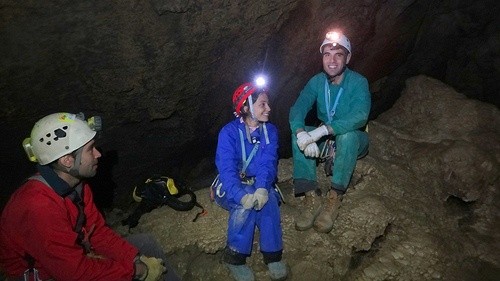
[267,261,287,280]
[312,189,341,234]
[295,190,322,231]
[225,262,255,281]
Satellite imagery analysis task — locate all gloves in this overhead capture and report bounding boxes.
[296,125,329,151]
[239,187,269,211]
[296,131,320,158]
[139,255,167,281]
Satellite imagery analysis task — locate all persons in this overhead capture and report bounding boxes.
[289,30,372,233]
[213,81,287,281]
[0,112,180,281]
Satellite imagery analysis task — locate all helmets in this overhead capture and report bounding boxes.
[232,81,269,116]
[21,112,103,166]
[319,29,352,58]
